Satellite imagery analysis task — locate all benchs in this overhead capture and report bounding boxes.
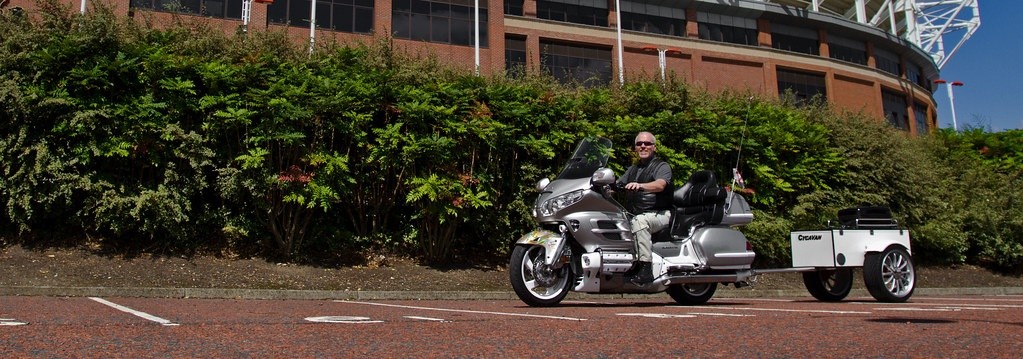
[823,206,898,229]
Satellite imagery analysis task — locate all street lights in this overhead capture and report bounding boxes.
[935,79,965,133]
[643,45,683,89]
[241,0,273,32]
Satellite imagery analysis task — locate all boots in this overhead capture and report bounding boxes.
[629,262,655,284]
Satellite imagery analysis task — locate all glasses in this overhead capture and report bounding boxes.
[635,141,653,146]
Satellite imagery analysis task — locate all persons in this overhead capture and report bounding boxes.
[610,131,676,284]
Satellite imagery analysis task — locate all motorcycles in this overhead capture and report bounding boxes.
[509,137,756,305]
